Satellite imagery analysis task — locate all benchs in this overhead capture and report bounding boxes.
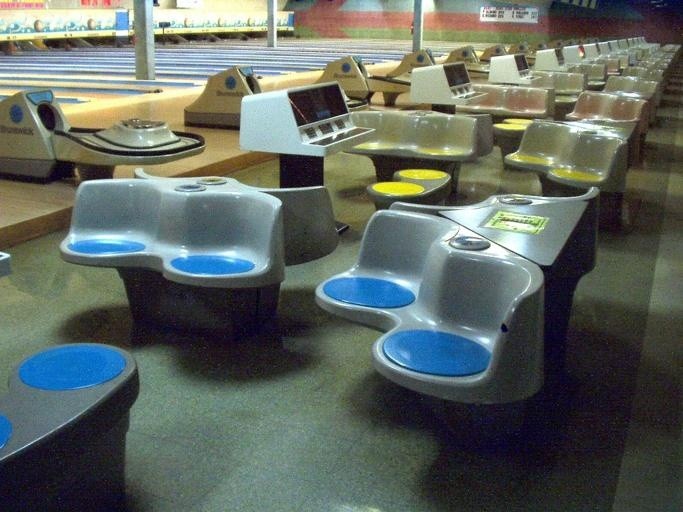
[1,339,139,510]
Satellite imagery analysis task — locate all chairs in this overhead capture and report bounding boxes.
[60,37,683,444]
